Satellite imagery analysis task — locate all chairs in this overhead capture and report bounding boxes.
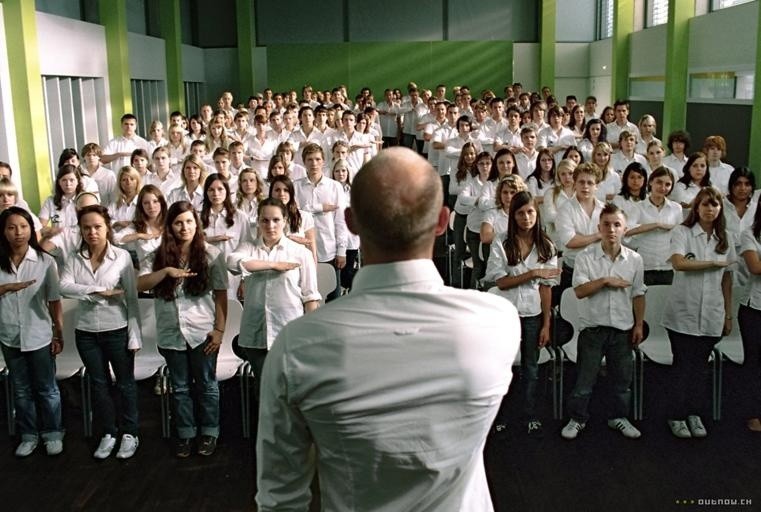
[443,172,761,432]
[0,250,363,437]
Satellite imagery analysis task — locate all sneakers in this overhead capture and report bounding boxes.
[527,417,544,439]
[116,433,140,459]
[607,416,642,439]
[561,418,586,439]
[687,416,707,437]
[748,418,761,432]
[16,441,38,457]
[94,433,117,459]
[44,440,63,455]
[492,421,506,441]
[197,435,217,456]
[174,437,192,458]
[668,416,691,438]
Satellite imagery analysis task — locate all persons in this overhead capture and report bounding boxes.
[1,83,760,460]
[252,146,523,512]
